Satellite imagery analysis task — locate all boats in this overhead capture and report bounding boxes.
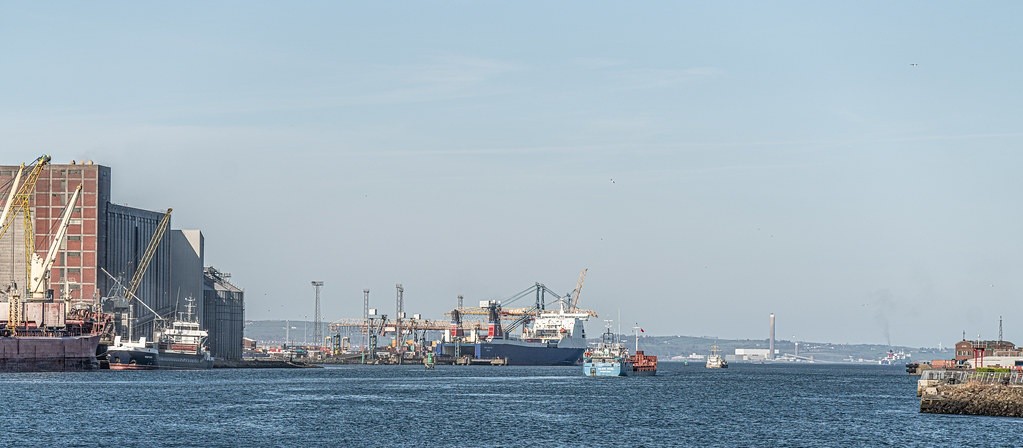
[881,347,912,365]
[436,266,599,367]
[0,155,215,373]
[624,322,657,377]
[904,314,1016,376]
[917,366,1023,398]
[953,345,1023,369]
[705,339,728,369]
[583,320,634,377]
